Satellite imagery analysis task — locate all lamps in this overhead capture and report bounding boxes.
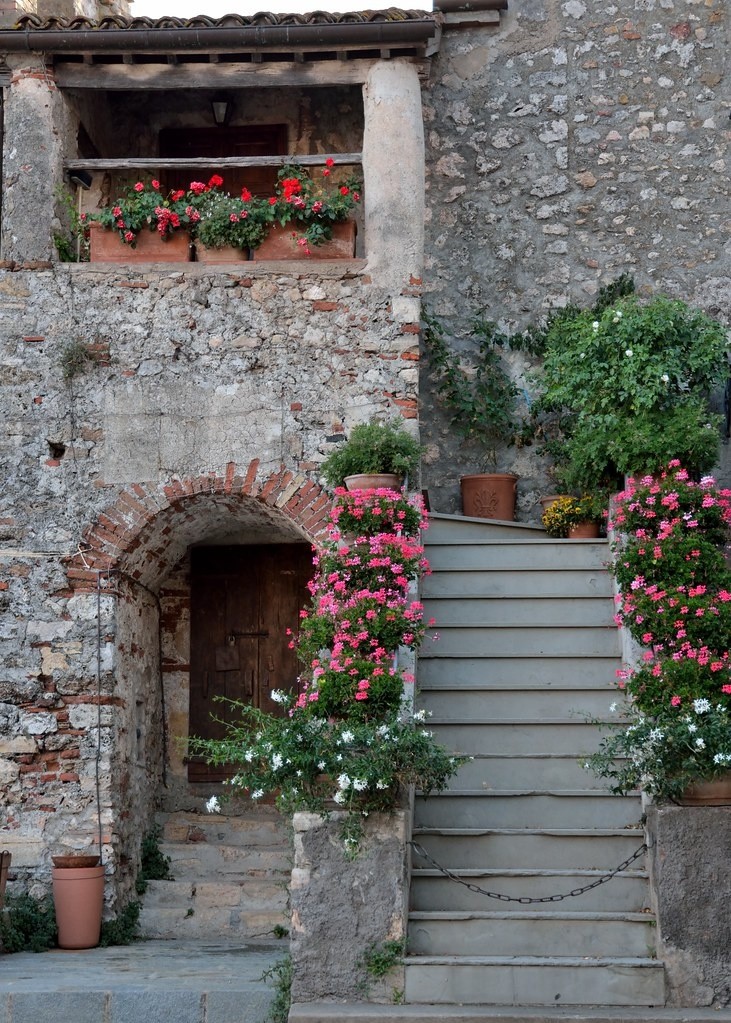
[210,90,234,127]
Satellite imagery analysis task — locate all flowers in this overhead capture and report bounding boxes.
[586,460,731,794]
[100,157,362,253]
[180,487,463,821]
[541,490,613,539]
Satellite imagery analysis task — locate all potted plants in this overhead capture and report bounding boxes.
[85,214,357,265]
[667,755,731,809]
[317,420,426,493]
[567,516,601,540]
[418,300,528,520]
[541,495,581,515]
[51,854,106,950]
